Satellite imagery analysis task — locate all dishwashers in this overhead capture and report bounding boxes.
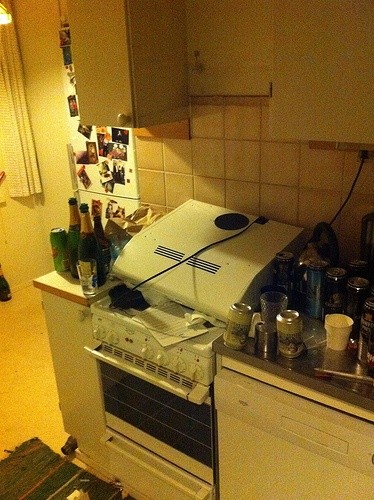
[212,347,374,499]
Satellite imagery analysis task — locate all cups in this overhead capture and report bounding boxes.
[324,314,354,351]
[75,257,99,295]
[246,312,262,338]
[261,292,288,346]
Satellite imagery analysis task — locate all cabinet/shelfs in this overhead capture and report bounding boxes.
[266,1,374,144]
[68,0,189,128]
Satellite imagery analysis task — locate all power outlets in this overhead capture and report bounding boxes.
[357,150,374,163]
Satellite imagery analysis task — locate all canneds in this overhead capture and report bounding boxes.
[276,310,304,359]
[49,227,70,271]
[223,302,253,349]
[274,252,374,342]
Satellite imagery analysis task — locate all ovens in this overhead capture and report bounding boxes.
[84,279,240,500]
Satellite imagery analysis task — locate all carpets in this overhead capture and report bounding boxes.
[0,437,135,499]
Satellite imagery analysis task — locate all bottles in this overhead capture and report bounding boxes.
[66,197,81,280]
[91,200,111,276]
[0,275,11,301]
[78,203,105,287]
